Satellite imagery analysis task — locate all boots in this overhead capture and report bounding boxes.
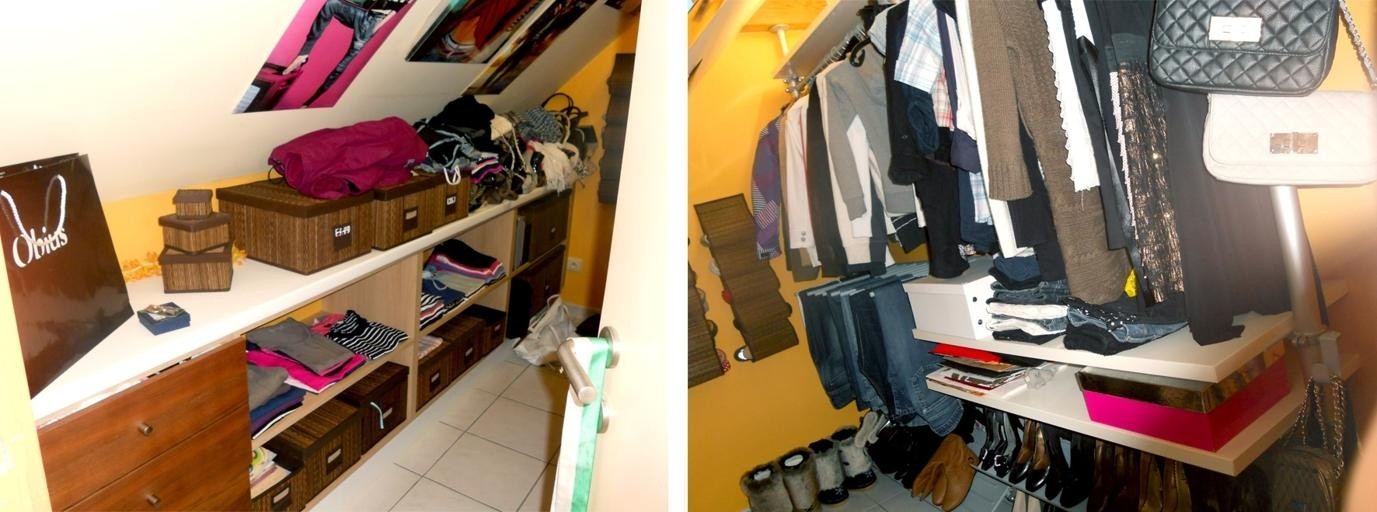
[740,461,795,512]
[777,446,824,512]
[807,438,850,508]
[829,425,878,493]
[911,433,980,512]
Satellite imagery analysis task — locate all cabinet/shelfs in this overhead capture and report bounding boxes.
[37,335,252,512]
[508,188,572,340]
[912,278,1359,477]
[245,210,517,512]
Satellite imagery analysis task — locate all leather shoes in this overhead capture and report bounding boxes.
[1086,441,1205,512]
[1011,489,1063,512]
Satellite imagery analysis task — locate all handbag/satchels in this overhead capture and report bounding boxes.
[0,152,136,400]
[1202,0,1376,188]
[1147,0,1342,98]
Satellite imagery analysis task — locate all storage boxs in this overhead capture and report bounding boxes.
[518,189,571,262]
[250,361,409,512]
[903,256,996,339]
[158,162,472,293]
[416,303,506,411]
[513,244,565,335]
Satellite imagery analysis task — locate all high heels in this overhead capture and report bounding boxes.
[976,408,1097,509]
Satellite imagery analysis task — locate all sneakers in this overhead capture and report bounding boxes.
[854,410,946,490]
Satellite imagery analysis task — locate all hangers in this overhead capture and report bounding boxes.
[808,0,892,85]
